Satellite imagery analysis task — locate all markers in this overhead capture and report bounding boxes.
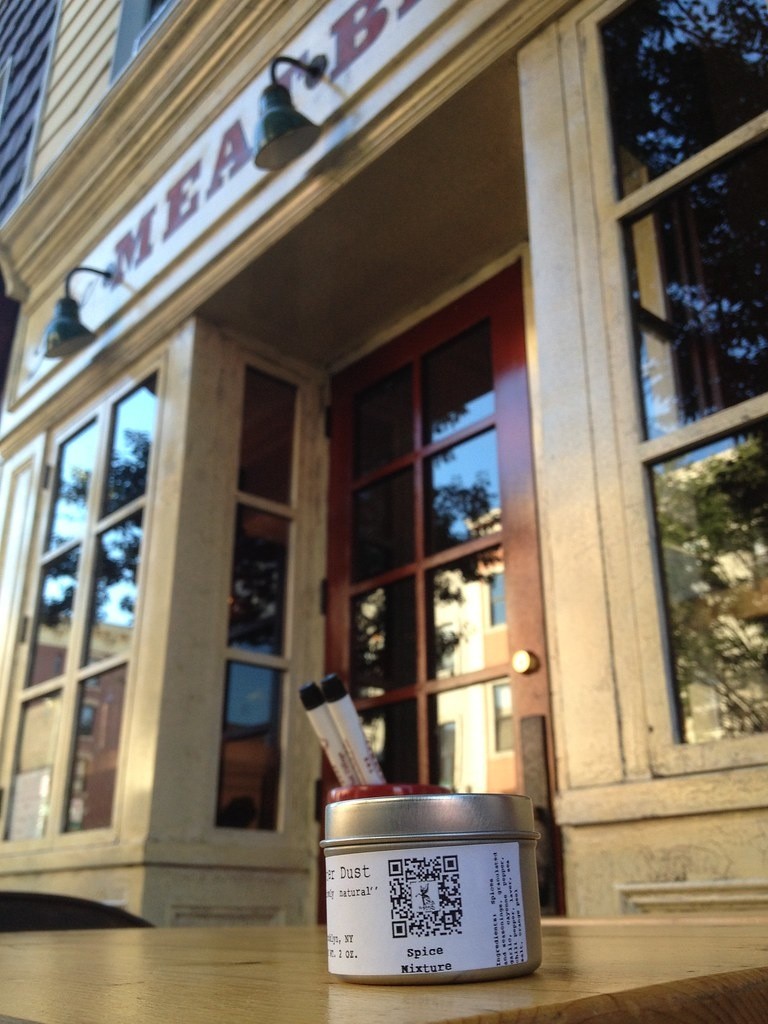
[321,674,390,786]
[298,681,359,786]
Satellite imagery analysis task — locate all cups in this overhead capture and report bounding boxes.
[329,785,448,802]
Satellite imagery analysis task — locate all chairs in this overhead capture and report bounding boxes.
[43,262,121,356]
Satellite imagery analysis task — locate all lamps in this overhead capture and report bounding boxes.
[253,54,328,167]
